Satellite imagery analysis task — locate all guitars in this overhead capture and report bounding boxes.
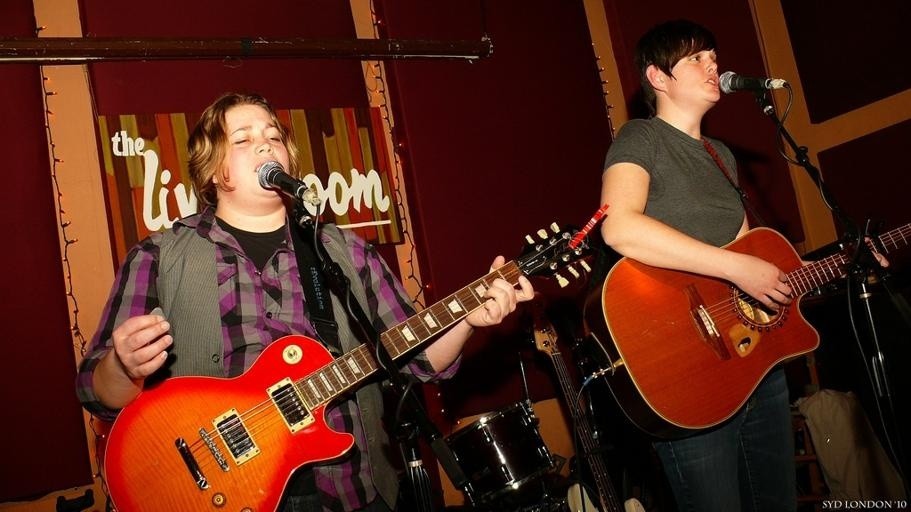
[526,291,646,512]
[104,205,608,512]
[583,223,910,441]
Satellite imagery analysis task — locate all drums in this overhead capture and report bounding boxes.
[443,399,555,507]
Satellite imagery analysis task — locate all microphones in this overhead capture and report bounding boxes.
[257,160,321,207]
[719,70,787,95]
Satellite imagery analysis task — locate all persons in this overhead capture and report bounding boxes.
[74,91,535,512]
[599,19,891,512]
